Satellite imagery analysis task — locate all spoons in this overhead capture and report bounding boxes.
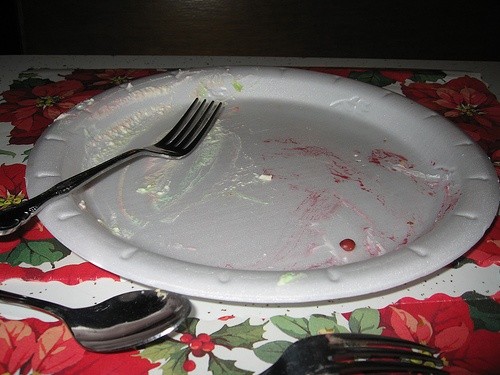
[0,287,191,353]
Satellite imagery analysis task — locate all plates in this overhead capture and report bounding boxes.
[25,64,500,304]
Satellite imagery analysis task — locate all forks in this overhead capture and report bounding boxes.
[257,332,452,375]
[0,98,223,236]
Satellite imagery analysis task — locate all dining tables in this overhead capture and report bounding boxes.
[0,55,500,374]
